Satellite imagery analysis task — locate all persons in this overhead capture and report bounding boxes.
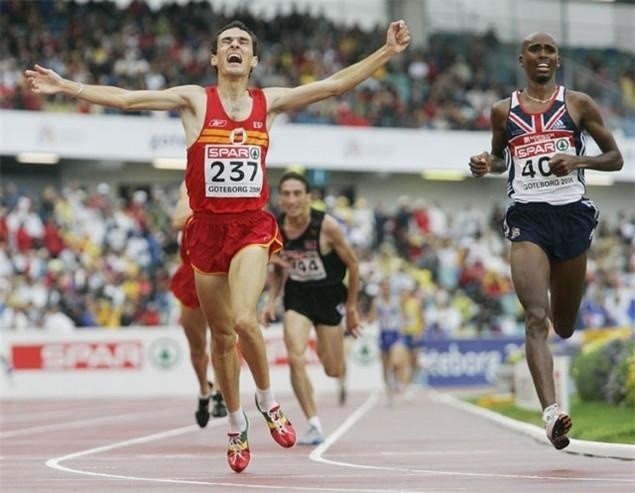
[465,29,625,450]
[22,17,414,474]
[1,0,635,407]
[173,173,364,445]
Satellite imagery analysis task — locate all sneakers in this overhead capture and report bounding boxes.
[227,410,250,472]
[297,423,325,445]
[255,392,296,448]
[543,406,572,450]
[211,389,227,417]
[195,381,213,427]
